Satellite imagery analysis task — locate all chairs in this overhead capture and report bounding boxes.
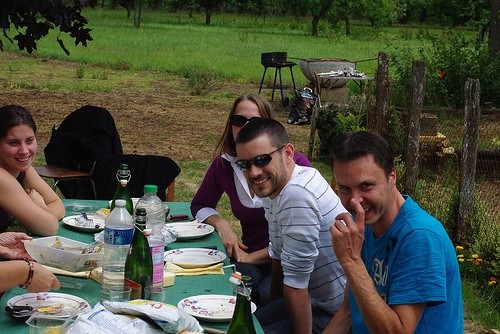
[32,105,110,199]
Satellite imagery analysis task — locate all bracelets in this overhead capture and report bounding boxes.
[18,257,34,290]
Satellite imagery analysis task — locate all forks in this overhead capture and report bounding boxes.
[81,211,93,224]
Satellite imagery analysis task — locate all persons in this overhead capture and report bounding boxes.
[190,93,311,307]
[235,118,353,334]
[0,105,65,236]
[0,232,60,293]
[322,130,464,334]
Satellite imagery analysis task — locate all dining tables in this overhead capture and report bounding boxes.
[0,199,265,334]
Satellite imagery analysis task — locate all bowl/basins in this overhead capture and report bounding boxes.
[22,235,106,273]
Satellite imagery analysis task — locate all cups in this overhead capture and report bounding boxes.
[109,286,132,303]
[25,307,76,334]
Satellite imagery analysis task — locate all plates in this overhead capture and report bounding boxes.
[165,221,215,240]
[162,248,227,269]
[7,291,91,319]
[177,294,258,322]
[62,214,106,232]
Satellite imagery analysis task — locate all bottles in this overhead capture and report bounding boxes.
[135,184,166,303]
[225,276,258,334]
[125,208,154,302]
[101,199,136,300]
[109,163,133,215]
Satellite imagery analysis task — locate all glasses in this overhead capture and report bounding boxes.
[230,115,259,127]
[235,146,285,171]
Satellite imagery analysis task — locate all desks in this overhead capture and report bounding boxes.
[308,71,374,162]
